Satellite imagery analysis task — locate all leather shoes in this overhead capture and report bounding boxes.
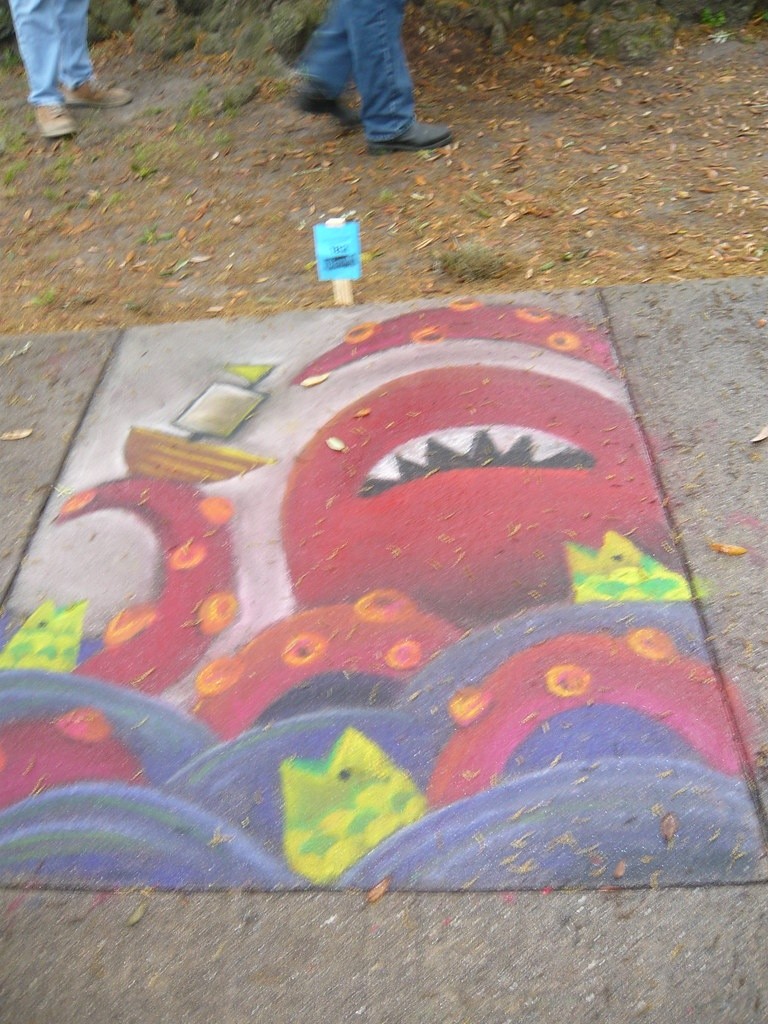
[300,90,364,128]
[366,120,453,151]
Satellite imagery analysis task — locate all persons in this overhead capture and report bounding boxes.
[7,0,132,138]
[292,0,452,152]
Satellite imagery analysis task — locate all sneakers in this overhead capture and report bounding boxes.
[61,76,134,107]
[33,103,79,137]
